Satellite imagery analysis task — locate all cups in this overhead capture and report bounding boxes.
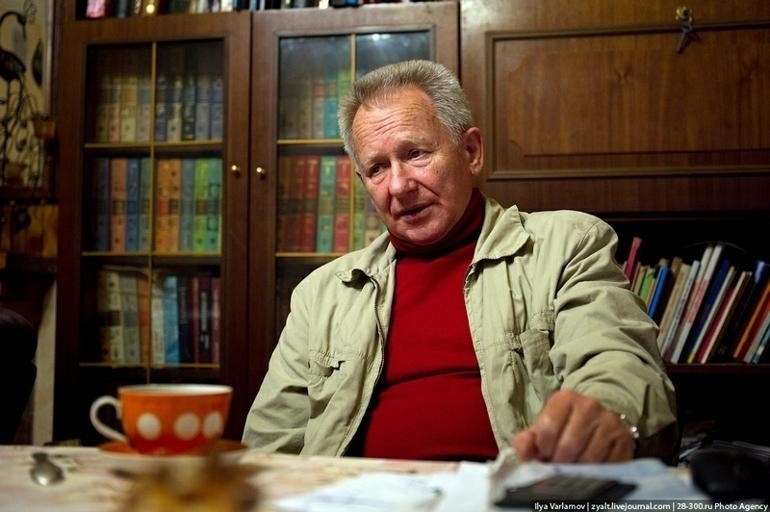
[89,382,232,453]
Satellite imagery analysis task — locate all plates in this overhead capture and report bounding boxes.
[97,435,253,474]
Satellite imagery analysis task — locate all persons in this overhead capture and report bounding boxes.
[240,59,679,465]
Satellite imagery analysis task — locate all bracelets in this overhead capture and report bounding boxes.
[607,408,639,440]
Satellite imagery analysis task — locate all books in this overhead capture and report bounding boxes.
[617,234,770,367]
[75,1,436,368]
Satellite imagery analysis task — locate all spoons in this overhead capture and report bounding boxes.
[26,444,64,490]
